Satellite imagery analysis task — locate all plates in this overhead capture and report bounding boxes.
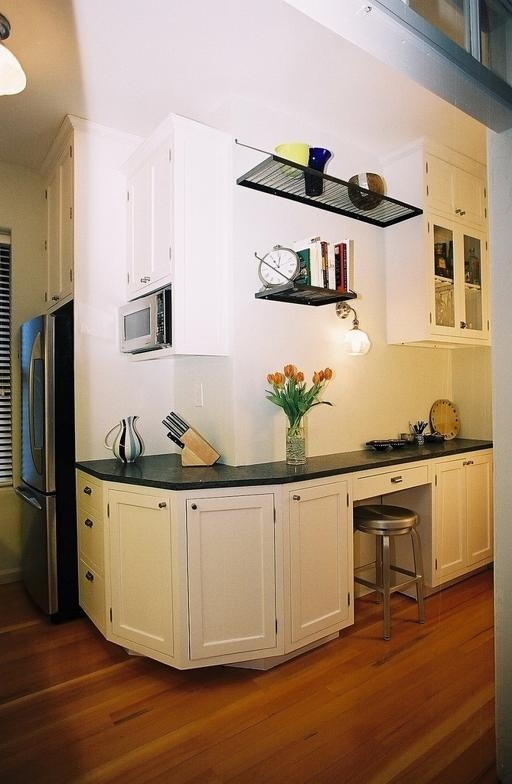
[432,399,461,441]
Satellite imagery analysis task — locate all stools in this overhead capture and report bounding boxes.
[352,504,426,641]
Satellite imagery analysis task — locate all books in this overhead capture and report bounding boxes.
[290,238,356,292]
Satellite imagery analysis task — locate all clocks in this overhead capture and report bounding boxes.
[257,243,302,288]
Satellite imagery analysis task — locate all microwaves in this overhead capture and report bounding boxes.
[118,287,172,353]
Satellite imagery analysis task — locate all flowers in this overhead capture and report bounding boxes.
[264,364,335,465]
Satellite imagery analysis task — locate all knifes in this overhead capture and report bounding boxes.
[162,432,184,448]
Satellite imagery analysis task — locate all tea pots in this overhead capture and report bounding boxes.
[104,415,143,464]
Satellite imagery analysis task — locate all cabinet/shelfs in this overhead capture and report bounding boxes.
[230,137,424,305]
[282,471,353,662]
[106,480,183,670]
[381,137,492,228]
[353,456,432,500]
[385,210,490,349]
[75,467,106,639]
[121,113,229,362]
[34,114,126,316]
[381,448,493,604]
[183,483,281,671]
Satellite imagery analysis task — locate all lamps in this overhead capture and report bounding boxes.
[335,301,371,356]
[0,13,27,98]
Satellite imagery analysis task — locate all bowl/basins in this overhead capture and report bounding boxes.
[389,439,406,449]
[366,440,389,451]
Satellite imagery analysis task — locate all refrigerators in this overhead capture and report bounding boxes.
[13,313,75,619]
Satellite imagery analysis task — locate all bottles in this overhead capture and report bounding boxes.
[435,240,453,278]
[465,245,480,285]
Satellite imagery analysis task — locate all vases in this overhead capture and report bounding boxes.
[304,148,332,196]
[285,417,307,465]
